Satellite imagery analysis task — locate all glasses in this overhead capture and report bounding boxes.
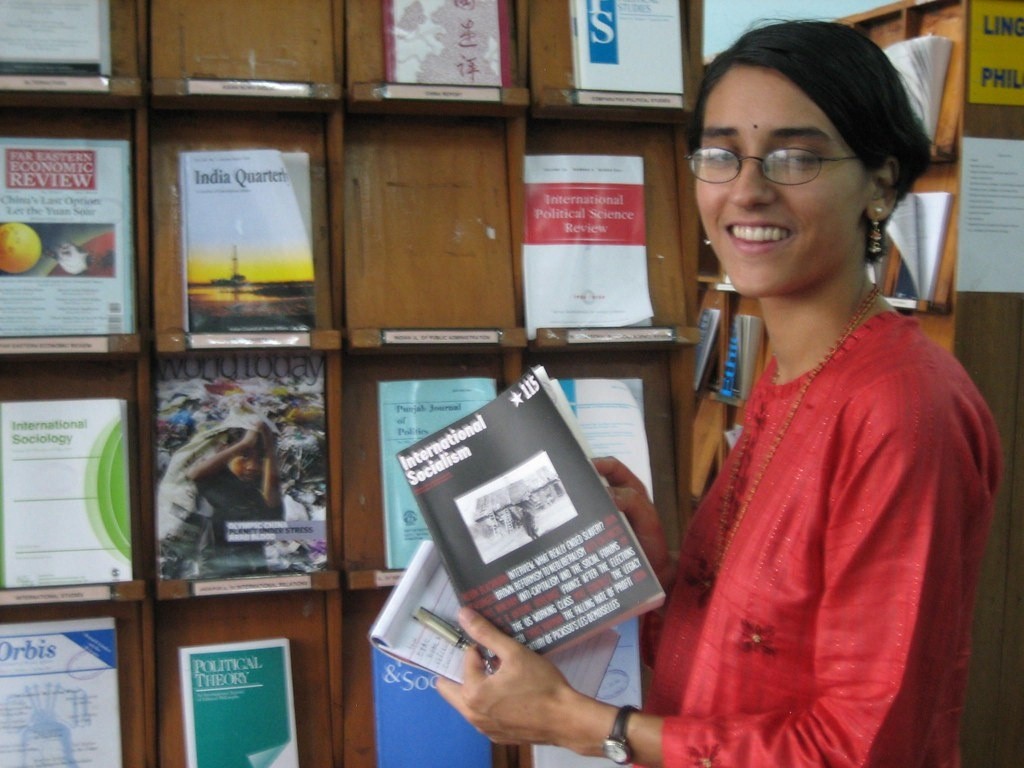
[682,144,859,185]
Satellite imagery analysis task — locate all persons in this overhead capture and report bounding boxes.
[434,22,1005,768]
[185,427,286,574]
[518,508,539,541]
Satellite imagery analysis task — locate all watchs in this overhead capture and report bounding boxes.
[603,705,643,765]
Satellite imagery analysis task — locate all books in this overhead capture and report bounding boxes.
[569,0,684,95]
[885,36,954,142]
[0,618,123,768]
[380,0,510,87]
[884,191,955,301]
[0,399,133,589]
[523,155,656,339]
[0,136,137,335]
[0,0,111,76]
[180,150,316,332]
[367,365,656,768]
[688,307,762,497]
[178,638,299,768]
[152,354,328,580]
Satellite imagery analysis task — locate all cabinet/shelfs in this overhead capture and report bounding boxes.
[0,0,1024,768]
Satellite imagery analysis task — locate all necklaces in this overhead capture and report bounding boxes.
[705,284,881,589]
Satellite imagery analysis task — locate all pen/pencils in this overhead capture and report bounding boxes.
[413,605,501,673]
